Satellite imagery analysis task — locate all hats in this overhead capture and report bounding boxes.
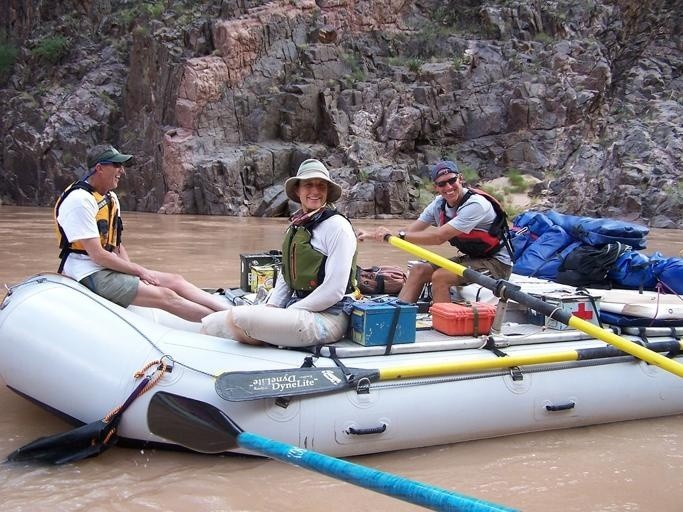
[285,159,342,202]
[88,148,133,168]
[431,161,458,181]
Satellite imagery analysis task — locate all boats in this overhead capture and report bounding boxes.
[0,264,682,460]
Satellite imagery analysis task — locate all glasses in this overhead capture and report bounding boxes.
[435,174,458,186]
[113,162,121,168]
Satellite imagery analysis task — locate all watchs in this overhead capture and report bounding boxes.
[398,231,406,241]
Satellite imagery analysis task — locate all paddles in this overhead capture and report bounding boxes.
[147,391,523,512]
[215,339,683,402]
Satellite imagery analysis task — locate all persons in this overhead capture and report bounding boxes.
[198,157,356,348]
[358,162,514,315]
[52,145,267,323]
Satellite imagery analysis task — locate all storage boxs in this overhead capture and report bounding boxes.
[425,299,500,339]
[238,253,283,295]
[538,290,602,334]
[249,264,280,294]
[344,296,421,347]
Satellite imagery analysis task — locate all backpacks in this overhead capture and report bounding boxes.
[557,241,628,290]
[357,266,406,295]
